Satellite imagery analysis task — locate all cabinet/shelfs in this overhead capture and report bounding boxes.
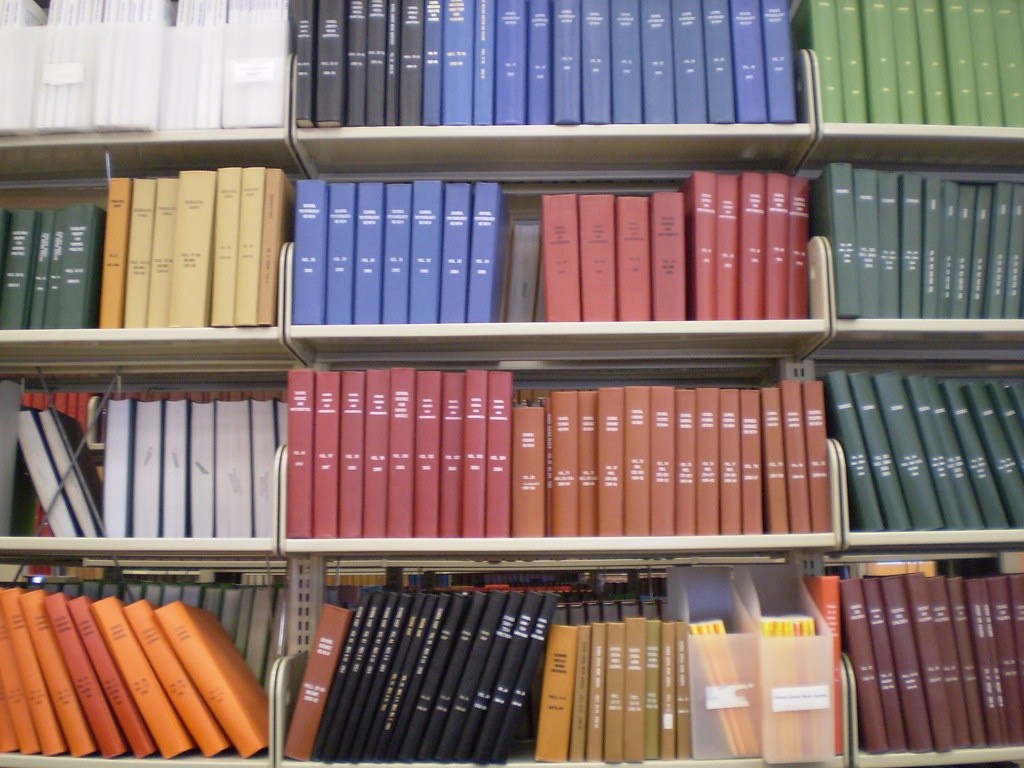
[0,48,1024,768]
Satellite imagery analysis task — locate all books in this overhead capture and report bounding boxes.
[1,161,1023,331]
[0,365,1024,539]
[0,0,1024,139]
[0,569,1024,767]
